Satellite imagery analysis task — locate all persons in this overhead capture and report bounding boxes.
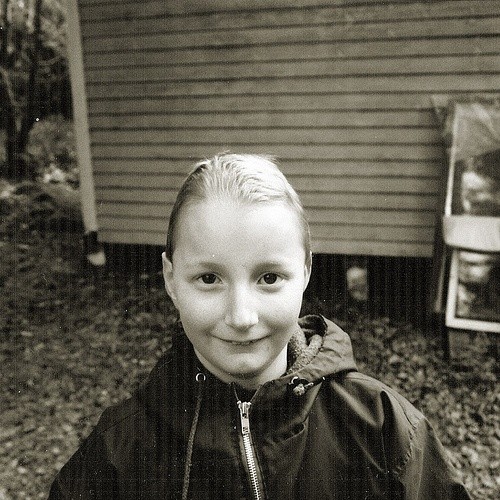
[47,152,480,500]
